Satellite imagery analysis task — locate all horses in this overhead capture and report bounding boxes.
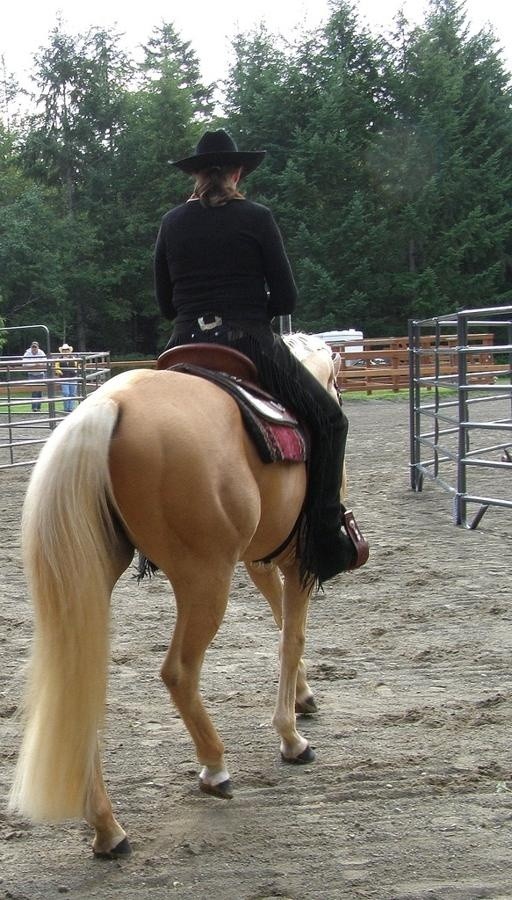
[0,328,347,861]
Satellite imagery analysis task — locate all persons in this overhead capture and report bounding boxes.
[21,341,48,412]
[153,131,356,580]
[54,343,78,412]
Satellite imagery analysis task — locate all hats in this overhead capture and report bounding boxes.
[167,129,266,180]
